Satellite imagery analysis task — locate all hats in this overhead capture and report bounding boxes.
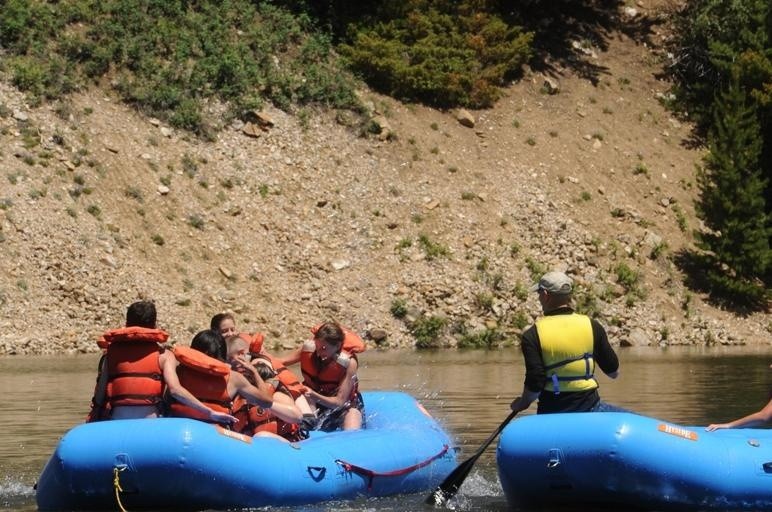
[530,271,573,295]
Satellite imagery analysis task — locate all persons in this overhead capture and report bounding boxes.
[509,268,630,417]
[278,321,367,433]
[86,299,242,428]
[704,397,772,432]
[162,312,320,451]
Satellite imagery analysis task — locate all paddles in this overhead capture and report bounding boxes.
[423,409,519,507]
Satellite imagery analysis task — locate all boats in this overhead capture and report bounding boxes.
[34,392,460,508]
[496,412,771,512]
